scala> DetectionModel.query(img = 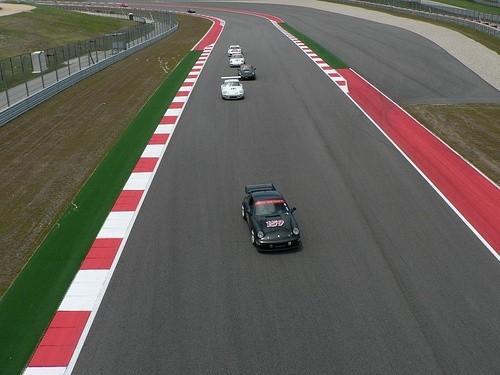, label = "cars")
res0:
[229,54,245,67]
[221,76,244,100]
[228,45,242,57]
[237,65,257,81]
[187,8,196,13]
[241,184,300,253]
[120,3,128,7]
